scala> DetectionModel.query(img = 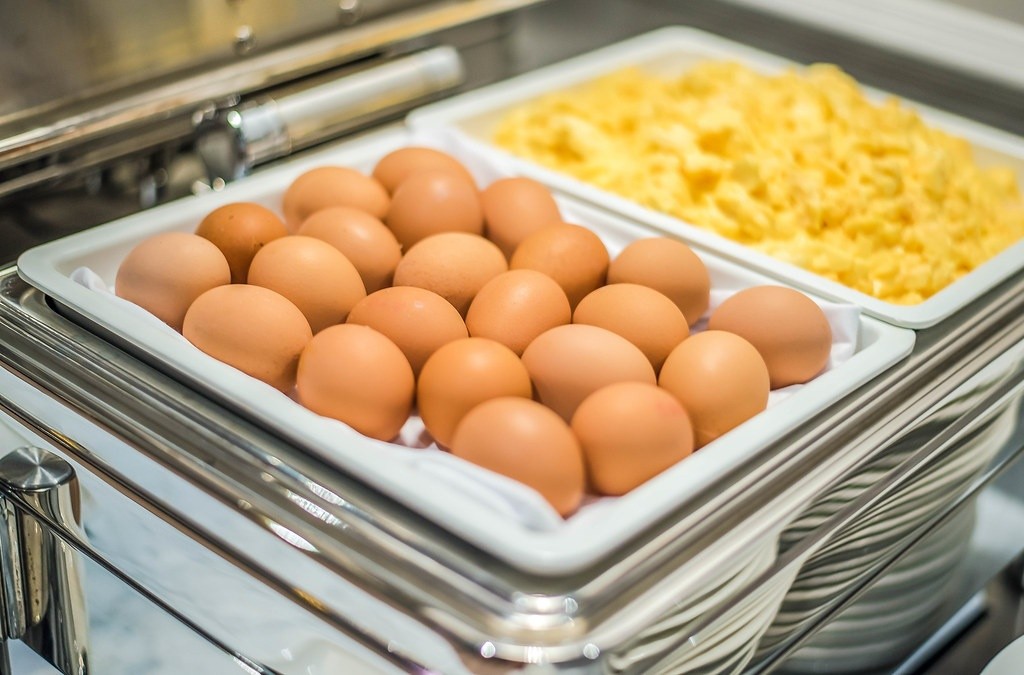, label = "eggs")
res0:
[115,147,832,520]
